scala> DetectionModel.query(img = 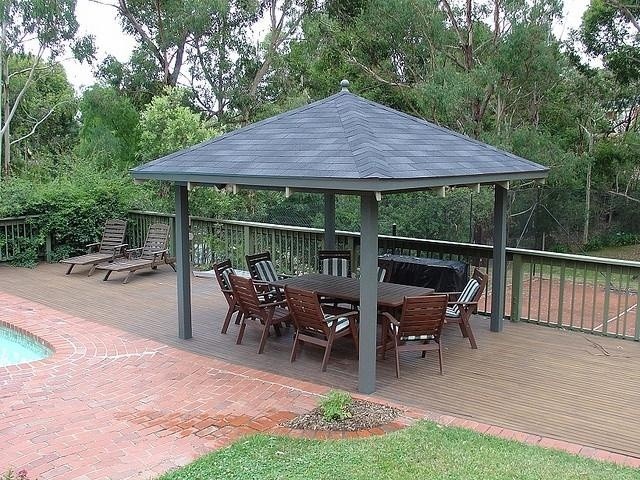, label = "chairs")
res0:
[59,219,177,284]
[213,249,489,379]
